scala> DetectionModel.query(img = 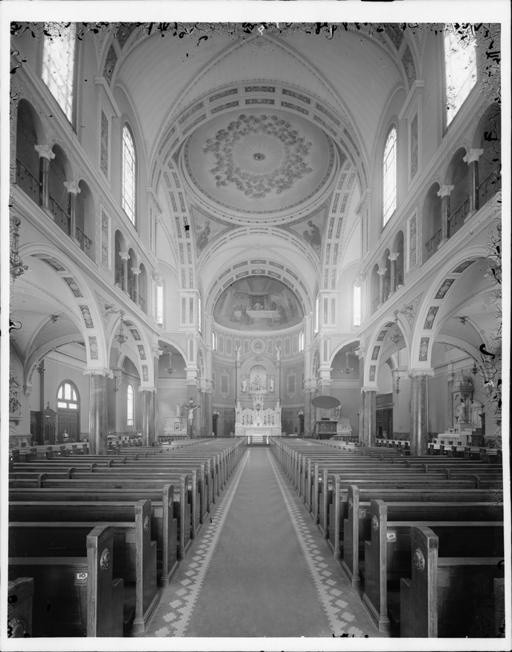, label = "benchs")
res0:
[7,473,192,560]
[8,500,162,634]
[8,524,125,637]
[9,432,246,540]
[7,485,178,588]
[399,526,504,635]
[329,473,504,558]
[269,435,502,540]
[343,484,504,588]
[363,498,504,631]
